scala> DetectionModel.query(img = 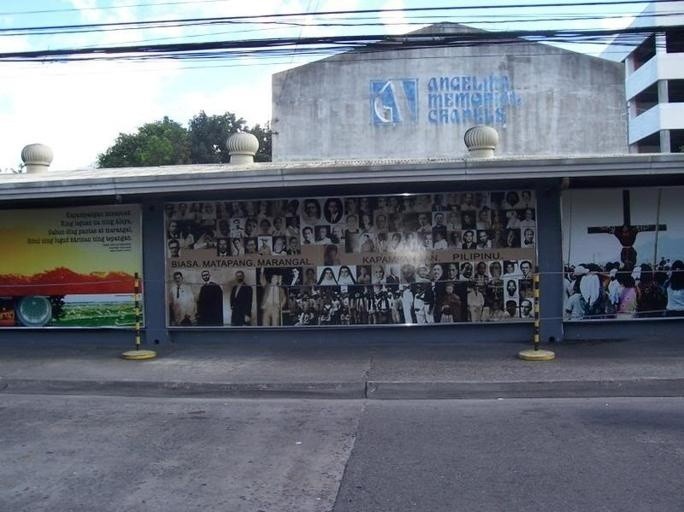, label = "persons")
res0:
[563,221,684,321]
